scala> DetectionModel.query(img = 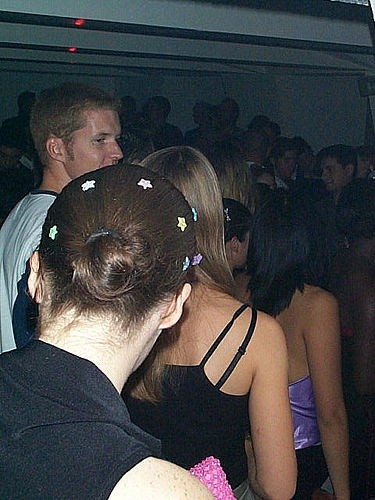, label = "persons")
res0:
[2,90,42,225]
[0,165,218,500]
[121,146,297,500]
[1,84,123,353]
[235,193,350,500]
[119,94,303,280]
[308,143,375,500]
